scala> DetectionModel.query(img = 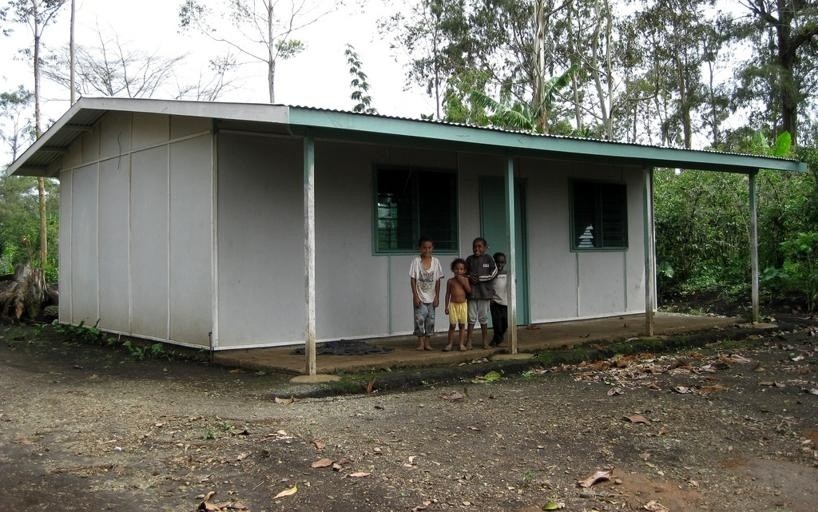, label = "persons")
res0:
[444,258,473,352]
[488,251,509,347]
[465,238,498,350]
[408,238,445,350]
